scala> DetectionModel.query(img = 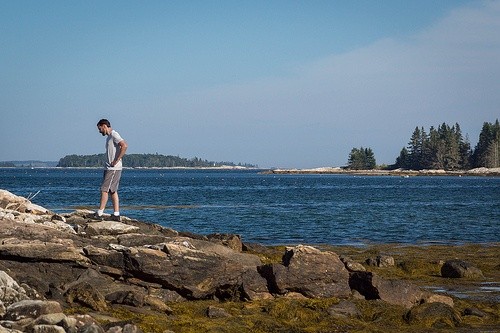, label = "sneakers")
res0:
[104,214,120,221]
[86,212,102,220]
